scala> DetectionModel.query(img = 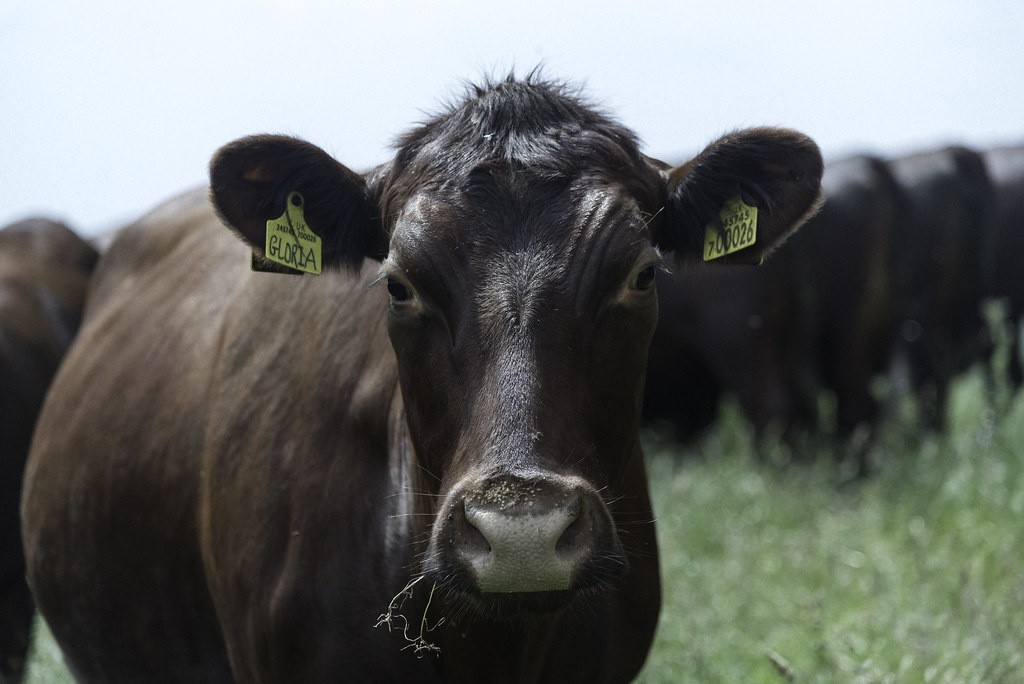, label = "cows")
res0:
[0,136,1023,683]
[19,75,827,683]
